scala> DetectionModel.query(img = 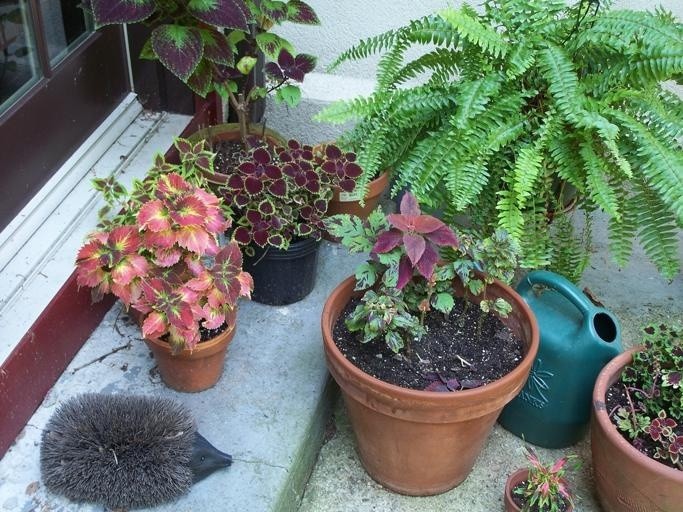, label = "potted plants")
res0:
[227,145,353,303]
[312,193,515,494]
[592,322,680,508]
[79,191,242,392]
[90,1,318,195]
[501,436,573,510]
[375,0,653,262]
